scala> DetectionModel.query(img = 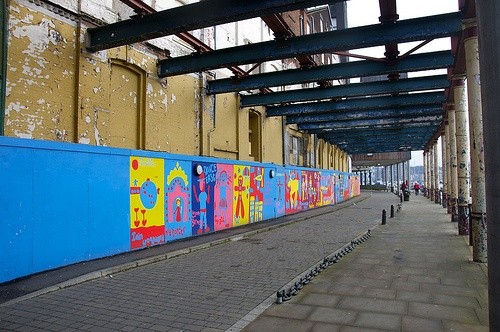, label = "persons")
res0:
[401,182,407,190]
[414,182,420,196]
